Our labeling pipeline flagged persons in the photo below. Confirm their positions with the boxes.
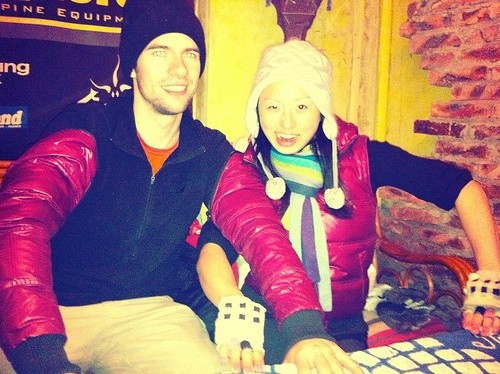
[1,1,362,374]
[195,37,500,374]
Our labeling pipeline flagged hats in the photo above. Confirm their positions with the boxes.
[118,0,209,84]
[247,40,347,209]
